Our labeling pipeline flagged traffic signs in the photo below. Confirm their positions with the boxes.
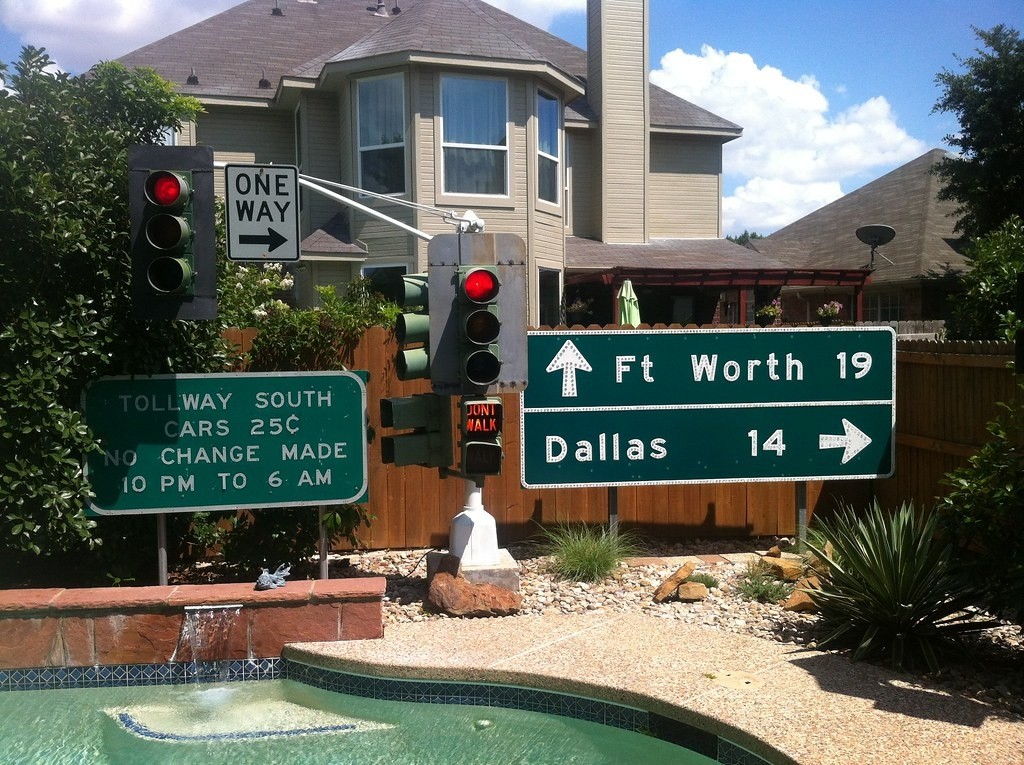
[520,408,897,491]
[520,330,895,406]
[224,162,301,264]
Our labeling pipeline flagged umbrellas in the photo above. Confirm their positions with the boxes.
[617,280,641,327]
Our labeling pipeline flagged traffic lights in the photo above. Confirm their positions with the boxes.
[379,392,452,468]
[142,166,197,300]
[454,264,501,387]
[392,273,433,381]
[458,395,506,479]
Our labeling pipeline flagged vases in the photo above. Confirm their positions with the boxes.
[819,317,833,327]
[758,315,770,327]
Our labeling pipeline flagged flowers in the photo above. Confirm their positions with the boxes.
[817,300,845,315]
[756,299,783,315]
